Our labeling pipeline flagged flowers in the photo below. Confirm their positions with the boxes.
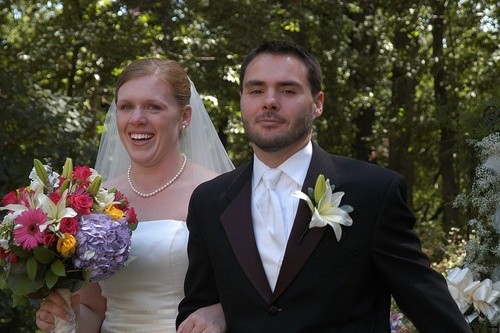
[288,174,355,243]
[0,155,140,332]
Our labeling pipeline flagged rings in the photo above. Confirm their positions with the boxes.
[38,310,43,319]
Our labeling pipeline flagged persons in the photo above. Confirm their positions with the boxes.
[175,38,471,333]
[34,58,236,333]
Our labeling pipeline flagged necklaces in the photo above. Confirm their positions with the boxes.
[127,153,187,198]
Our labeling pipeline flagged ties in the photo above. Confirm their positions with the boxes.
[258,168,287,293]
